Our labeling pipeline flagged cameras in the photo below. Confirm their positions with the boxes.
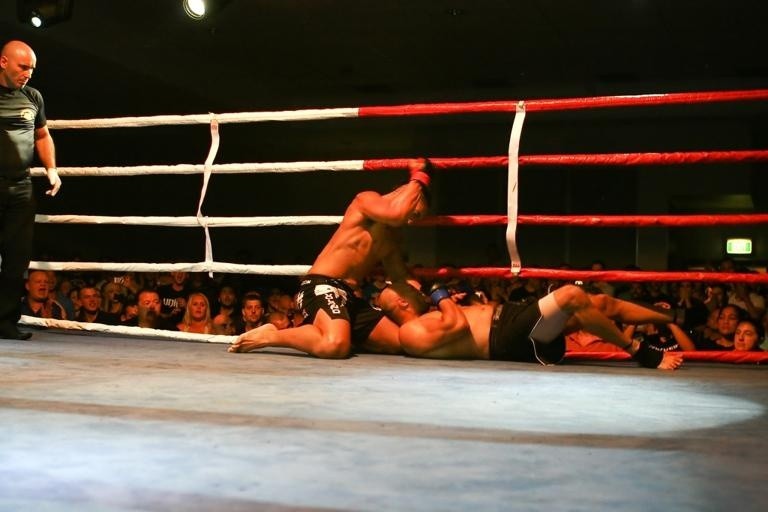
[114,277,124,283]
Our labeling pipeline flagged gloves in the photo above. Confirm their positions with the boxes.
[45,168,62,197]
[423,279,449,306]
[410,157,433,191]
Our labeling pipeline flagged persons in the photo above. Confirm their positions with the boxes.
[225,157,434,362]
[377,279,685,372]
[0,36,62,342]
[0,254,767,354]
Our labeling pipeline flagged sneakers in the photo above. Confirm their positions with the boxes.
[0,329,33,340]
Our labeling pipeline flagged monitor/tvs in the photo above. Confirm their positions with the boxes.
[726,238,753,255]
